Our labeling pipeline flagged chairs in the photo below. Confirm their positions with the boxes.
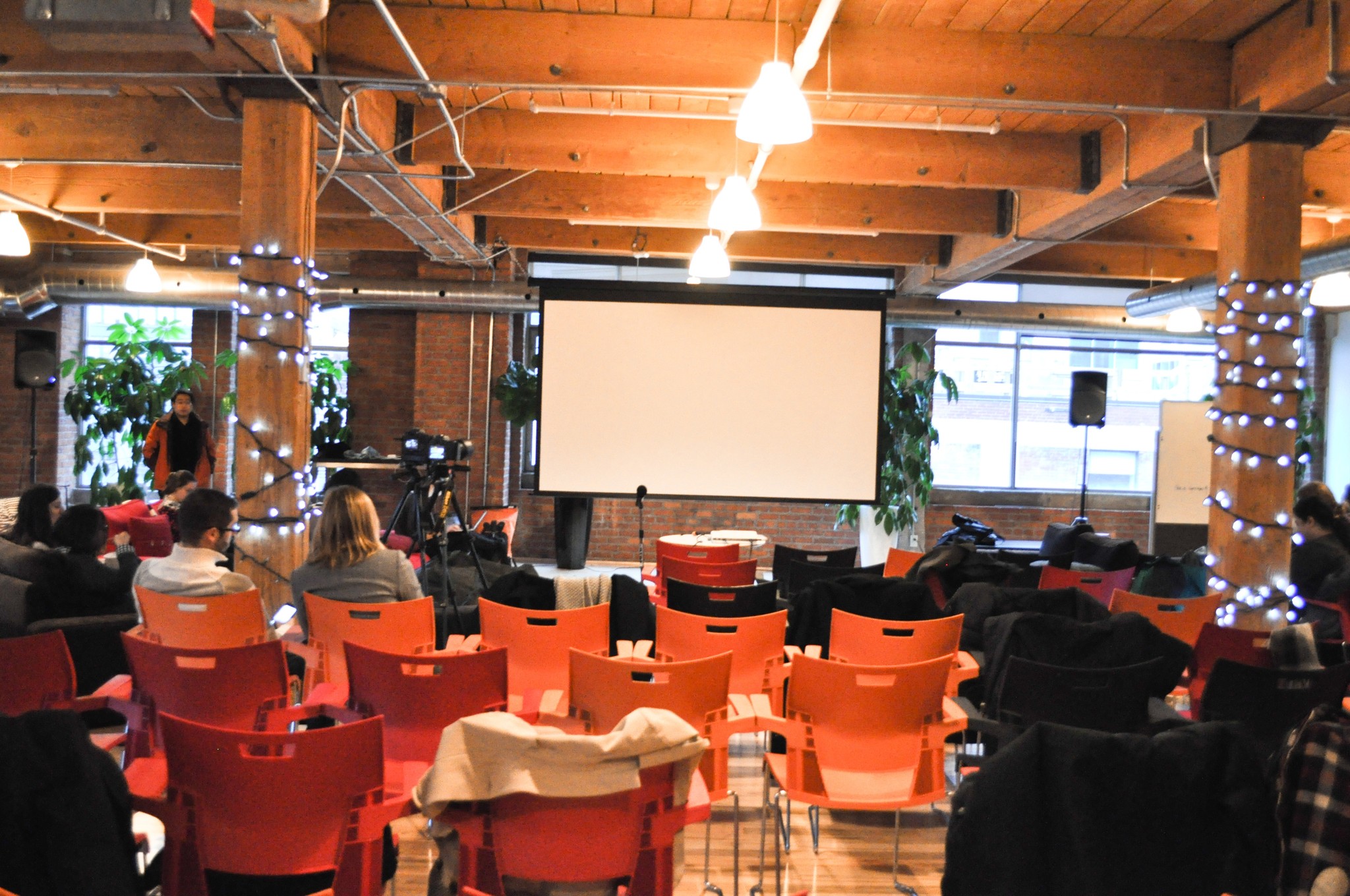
[472,504,522,570]
[1,537,1349,896]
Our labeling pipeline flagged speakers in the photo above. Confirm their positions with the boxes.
[13,328,57,390]
[1068,371,1108,429]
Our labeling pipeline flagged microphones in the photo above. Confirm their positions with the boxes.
[633,485,648,507]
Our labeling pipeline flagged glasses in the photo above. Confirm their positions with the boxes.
[220,524,242,535]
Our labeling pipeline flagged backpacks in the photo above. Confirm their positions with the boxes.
[0,706,148,896]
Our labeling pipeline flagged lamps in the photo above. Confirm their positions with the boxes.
[708,97,765,234]
[126,241,163,293]
[0,158,38,256]
[733,1,814,145]
[689,176,732,279]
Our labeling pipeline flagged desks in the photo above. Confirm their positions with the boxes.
[311,455,461,530]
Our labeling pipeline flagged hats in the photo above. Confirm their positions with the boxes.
[323,467,362,493]
[54,505,109,555]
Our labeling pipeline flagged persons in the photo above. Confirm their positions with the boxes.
[288,488,426,648]
[127,488,281,647]
[49,503,145,624]
[0,485,65,551]
[1290,480,1349,645]
[147,389,219,499]
[1,718,144,894]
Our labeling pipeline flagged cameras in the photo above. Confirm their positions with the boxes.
[397,430,449,465]
[428,438,474,459]
